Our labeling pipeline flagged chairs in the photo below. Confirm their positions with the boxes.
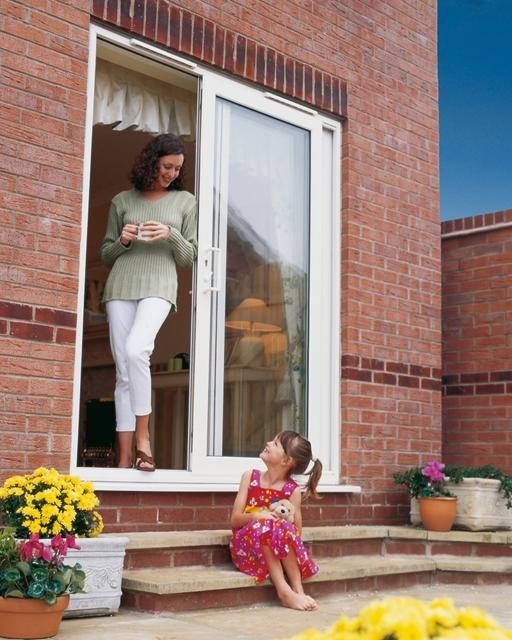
[81,396,120,467]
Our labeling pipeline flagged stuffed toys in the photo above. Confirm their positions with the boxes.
[249,500,296,525]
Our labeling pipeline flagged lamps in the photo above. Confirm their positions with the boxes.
[223,297,285,368]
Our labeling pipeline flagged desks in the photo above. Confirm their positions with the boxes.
[148,367,286,470]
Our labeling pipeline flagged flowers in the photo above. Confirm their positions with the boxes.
[0,463,103,604]
[292,595,511,640]
[420,460,446,496]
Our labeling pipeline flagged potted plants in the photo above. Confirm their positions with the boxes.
[393,461,512,530]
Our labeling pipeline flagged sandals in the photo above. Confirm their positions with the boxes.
[134,445,157,472]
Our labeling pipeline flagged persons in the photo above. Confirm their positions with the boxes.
[96,132,198,473]
[229,427,324,612]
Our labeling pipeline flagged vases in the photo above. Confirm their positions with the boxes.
[0,595,69,640]
[419,495,457,532]
[10,537,130,620]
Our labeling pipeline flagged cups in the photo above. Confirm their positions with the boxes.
[136,223,154,242]
